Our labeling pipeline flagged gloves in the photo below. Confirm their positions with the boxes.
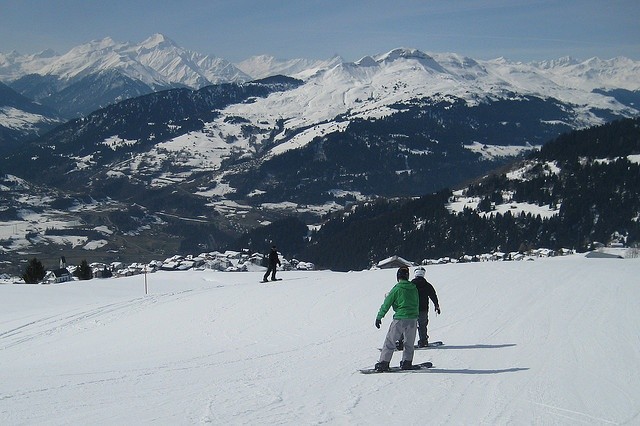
[375,319,382,329]
[434,304,440,313]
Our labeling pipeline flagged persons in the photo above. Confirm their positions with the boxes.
[263,246,281,282]
[399,266,440,349]
[375,267,419,372]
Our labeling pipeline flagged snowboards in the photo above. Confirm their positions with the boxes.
[259,279,282,283]
[377,341,443,351]
[359,362,433,374]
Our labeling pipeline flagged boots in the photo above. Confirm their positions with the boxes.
[418,339,428,348]
[399,360,420,370]
[375,360,390,371]
[397,340,403,349]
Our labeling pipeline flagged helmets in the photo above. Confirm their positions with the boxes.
[396,265,409,282]
[414,266,426,277]
